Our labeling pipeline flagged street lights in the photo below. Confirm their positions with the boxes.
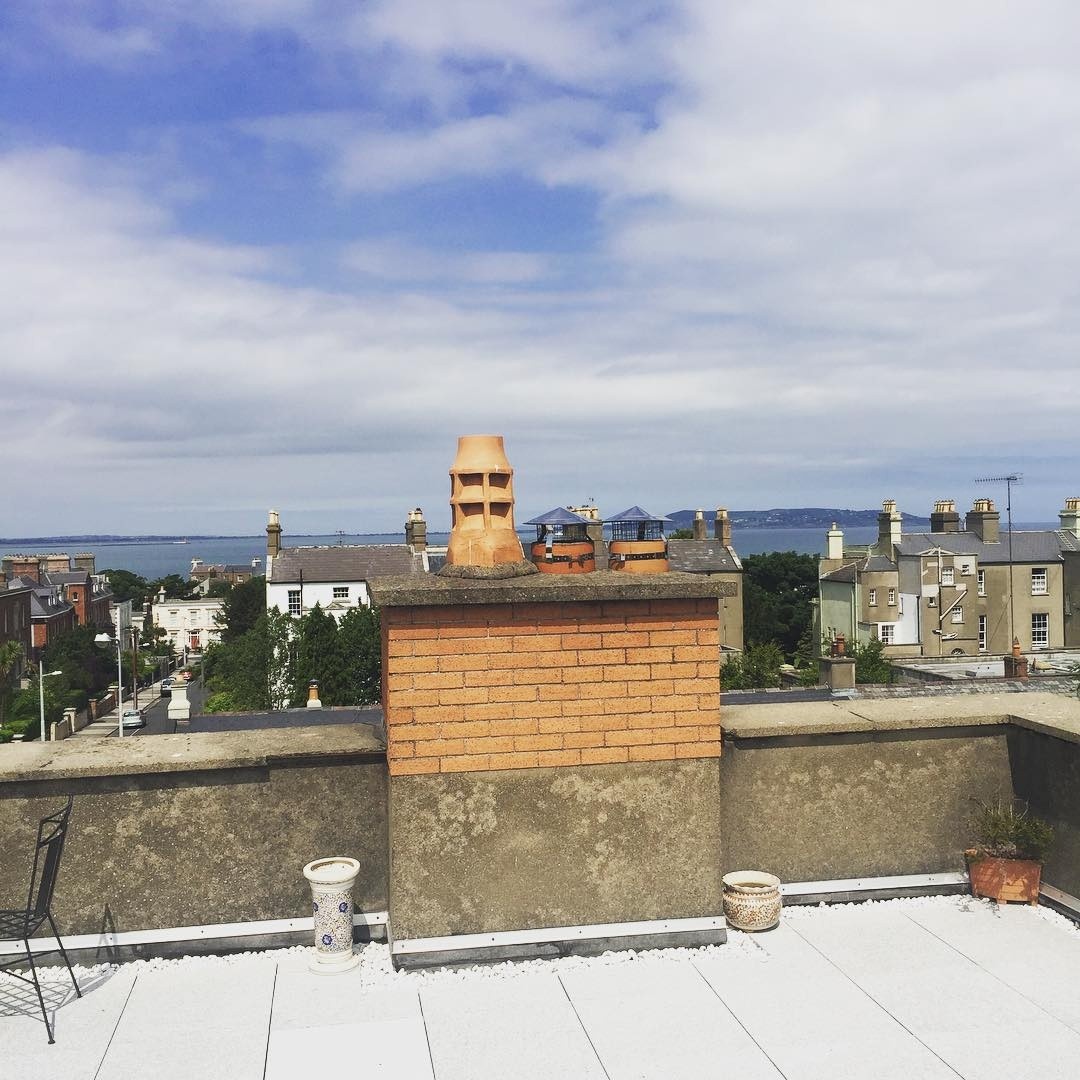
[133,643,150,709]
[94,632,123,737]
[184,617,191,666]
[40,660,62,741]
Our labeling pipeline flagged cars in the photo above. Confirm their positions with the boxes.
[123,710,147,728]
[184,671,191,679]
[161,676,175,690]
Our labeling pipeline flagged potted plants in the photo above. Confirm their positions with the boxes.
[964,795,1056,907]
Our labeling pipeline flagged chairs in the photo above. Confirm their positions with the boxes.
[0,794,82,1044]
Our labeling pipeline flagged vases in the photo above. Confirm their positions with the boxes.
[721,870,782,933]
[303,857,361,976]
